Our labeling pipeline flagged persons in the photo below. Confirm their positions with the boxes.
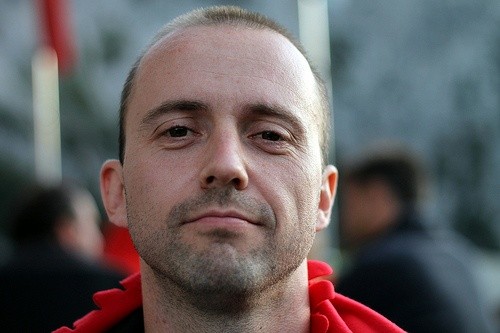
[0,183,134,333]
[53,4,409,333]
[331,147,490,332]
